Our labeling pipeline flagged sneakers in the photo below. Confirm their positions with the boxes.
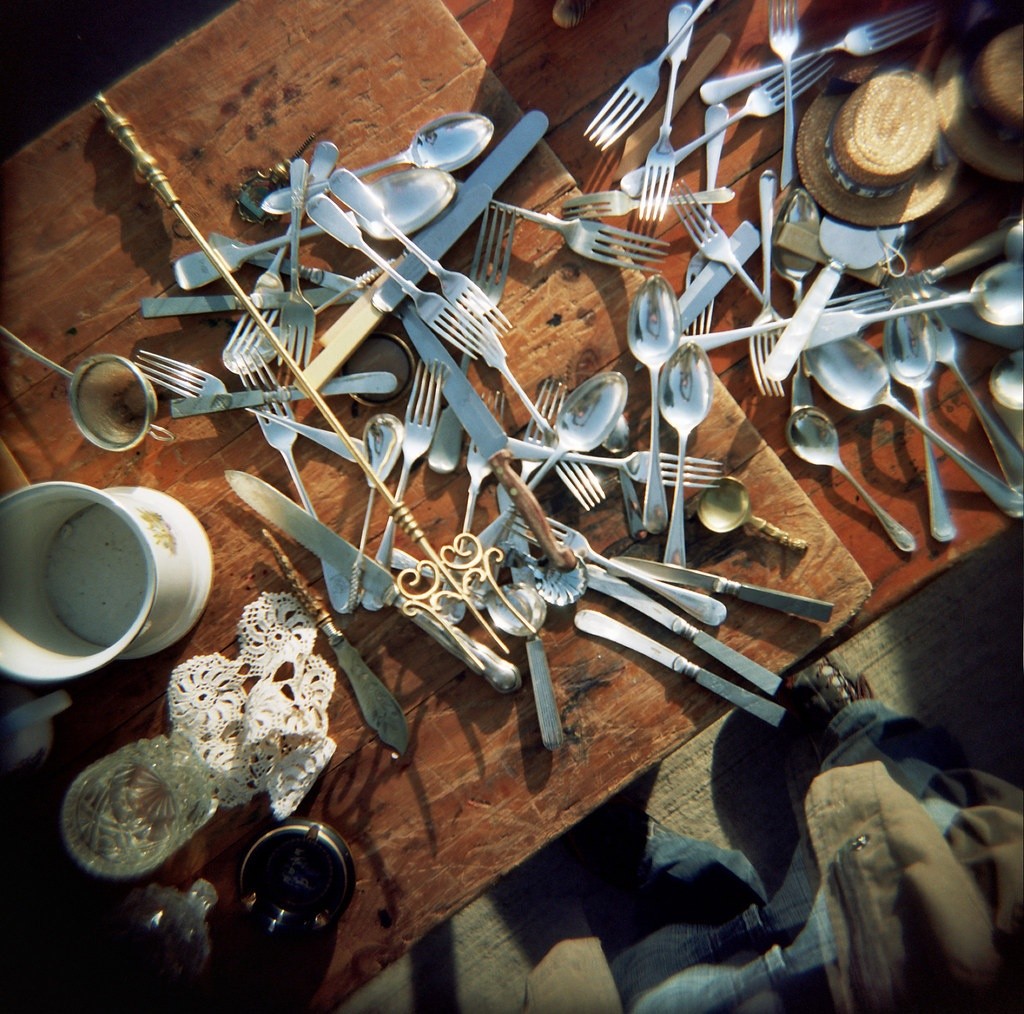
[764,655,872,744]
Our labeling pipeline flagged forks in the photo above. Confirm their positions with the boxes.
[133,0,1023,754]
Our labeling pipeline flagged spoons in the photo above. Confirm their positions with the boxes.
[658,338,715,574]
[623,275,681,536]
[805,338,1024,517]
[261,108,495,218]
[784,406,915,555]
[880,295,956,543]
[380,546,547,641]
[174,172,453,293]
[455,369,630,580]
[801,262,1024,346]
[340,413,406,615]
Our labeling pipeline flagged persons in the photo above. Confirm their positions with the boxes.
[525,646,1024,1012]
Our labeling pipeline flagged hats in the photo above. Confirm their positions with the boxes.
[933,24,1024,182]
[795,65,960,226]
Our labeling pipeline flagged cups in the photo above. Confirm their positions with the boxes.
[0,481,212,682]
[113,881,218,990]
[236,821,353,937]
[62,735,213,877]
